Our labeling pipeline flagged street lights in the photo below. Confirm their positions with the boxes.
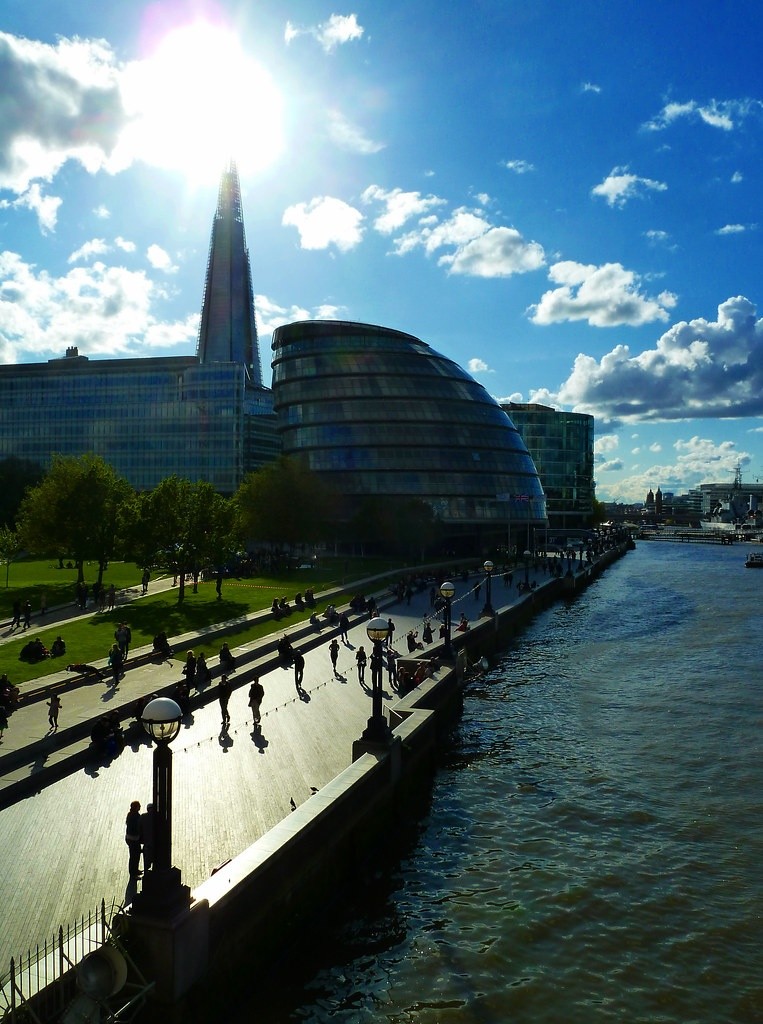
[439,581,455,653]
[586,539,592,562]
[578,541,584,568]
[523,550,530,586]
[139,697,184,895]
[483,561,493,610]
[366,618,389,729]
[595,526,627,552]
[566,544,573,572]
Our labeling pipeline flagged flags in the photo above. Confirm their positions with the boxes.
[496,492,529,505]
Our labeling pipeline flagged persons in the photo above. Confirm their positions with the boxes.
[277,634,294,662]
[272,596,292,615]
[11,588,48,630]
[171,682,192,715]
[304,589,315,604]
[109,620,132,684]
[296,592,305,608]
[0,673,19,738]
[249,677,264,725]
[126,801,154,880]
[59,552,204,615]
[220,642,235,673]
[323,548,593,698]
[217,673,233,724]
[91,709,124,753]
[293,649,304,687]
[185,649,196,684]
[132,693,157,725]
[66,663,106,679]
[153,631,175,657]
[46,693,62,728]
[310,611,322,632]
[20,636,66,660]
[197,652,211,682]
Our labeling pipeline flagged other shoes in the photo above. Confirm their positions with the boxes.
[253,717,261,725]
[137,869,143,874]
[221,716,230,724]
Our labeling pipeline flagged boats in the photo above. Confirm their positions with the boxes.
[744,552,763,568]
[699,458,763,532]
